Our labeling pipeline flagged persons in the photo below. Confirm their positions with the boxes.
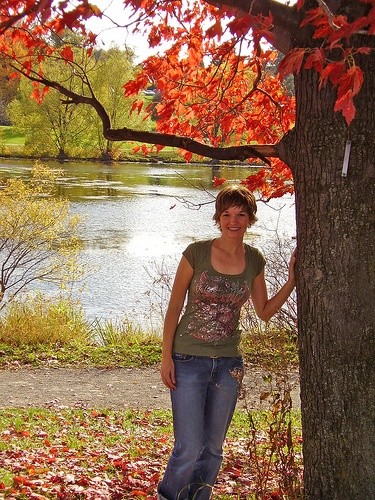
[157,186,300,500]
